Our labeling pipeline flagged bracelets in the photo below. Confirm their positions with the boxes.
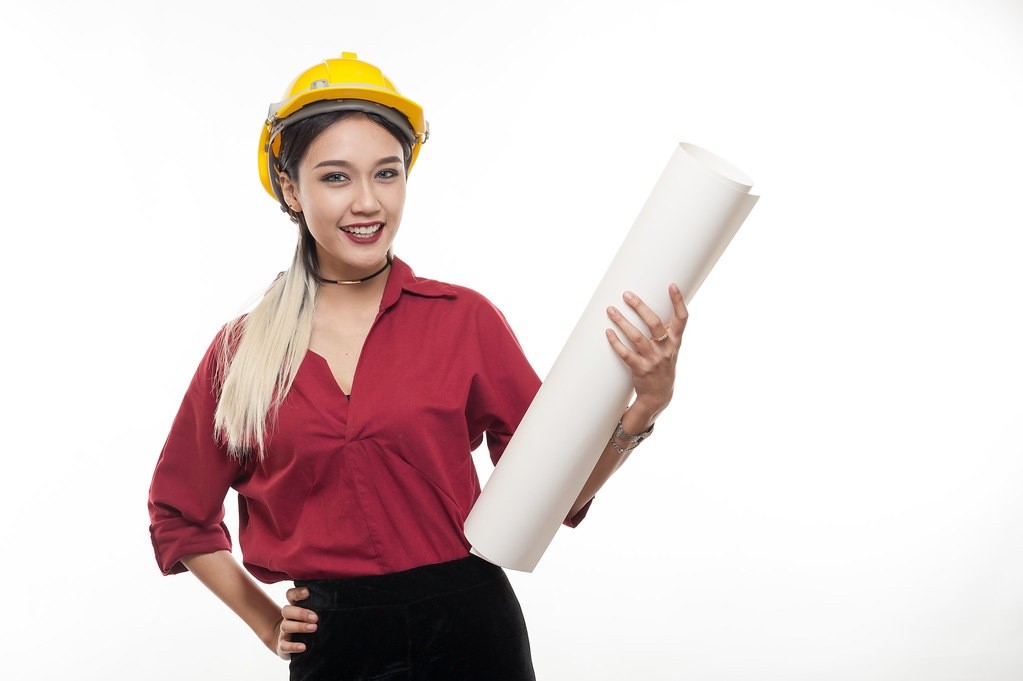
[614,411,654,443]
[610,438,640,453]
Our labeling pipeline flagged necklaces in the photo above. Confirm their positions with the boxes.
[316,260,390,285]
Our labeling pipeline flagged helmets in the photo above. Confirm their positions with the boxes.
[257,52,425,202]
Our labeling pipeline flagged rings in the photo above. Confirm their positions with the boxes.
[652,333,668,342]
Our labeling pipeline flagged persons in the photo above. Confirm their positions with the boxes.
[149,55,688,681]
